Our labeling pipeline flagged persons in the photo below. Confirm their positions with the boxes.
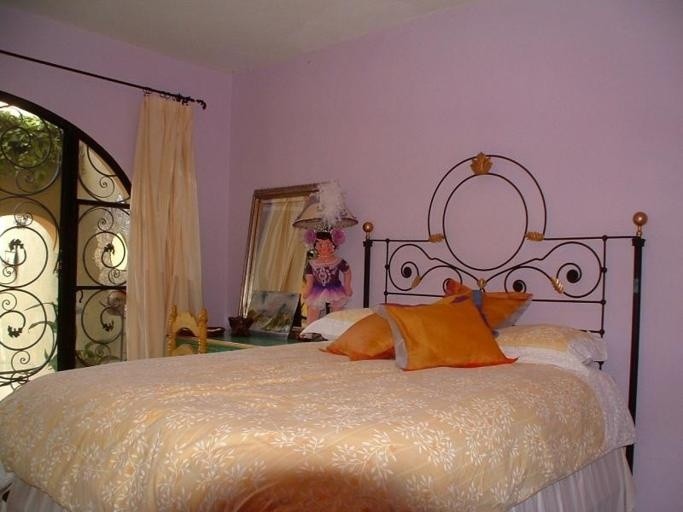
[301,228,352,325]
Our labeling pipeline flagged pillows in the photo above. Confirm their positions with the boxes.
[374,298,519,372]
[327,312,396,361]
[494,324,607,372]
[434,279,532,328]
[300,307,375,342]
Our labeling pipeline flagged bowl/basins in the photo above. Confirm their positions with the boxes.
[229,317,248,332]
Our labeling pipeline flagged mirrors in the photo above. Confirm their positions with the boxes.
[237,181,318,339]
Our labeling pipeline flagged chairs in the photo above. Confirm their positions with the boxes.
[163,303,210,356]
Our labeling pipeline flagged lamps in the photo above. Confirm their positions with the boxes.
[290,181,357,238]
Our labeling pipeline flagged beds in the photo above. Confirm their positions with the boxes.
[0,149,647,512]
